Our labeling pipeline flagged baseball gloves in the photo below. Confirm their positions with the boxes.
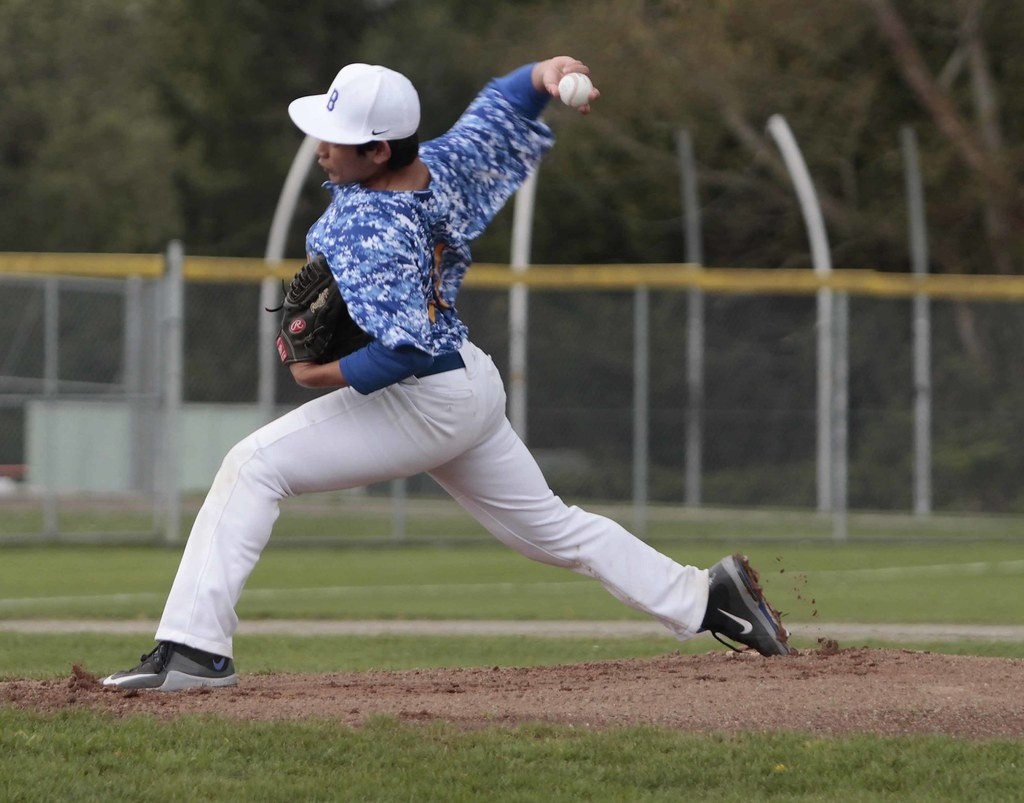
[275,254,349,366]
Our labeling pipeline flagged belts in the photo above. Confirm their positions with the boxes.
[413,351,465,379]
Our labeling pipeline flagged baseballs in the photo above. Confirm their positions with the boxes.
[559,72,594,108]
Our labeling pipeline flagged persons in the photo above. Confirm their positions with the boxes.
[102,54,788,693]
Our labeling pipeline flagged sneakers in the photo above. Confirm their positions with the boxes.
[96,641,237,691]
[696,553,792,656]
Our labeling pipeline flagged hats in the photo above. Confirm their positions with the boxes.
[288,62,420,145]
